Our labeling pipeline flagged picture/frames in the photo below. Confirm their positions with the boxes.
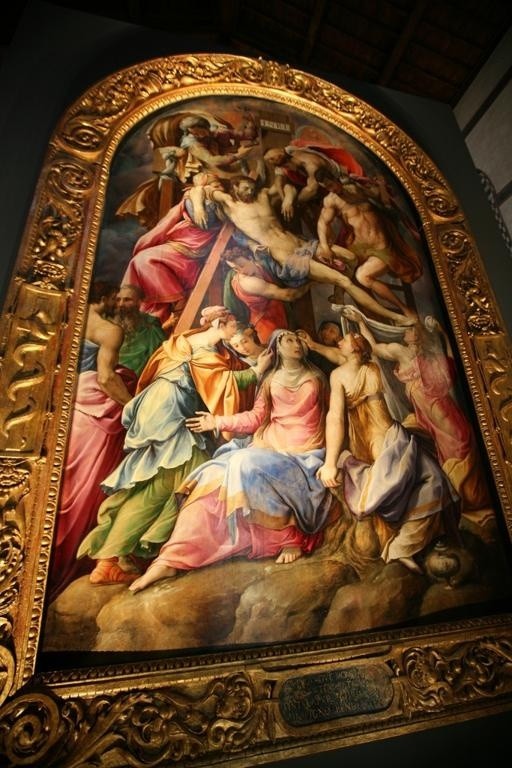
[0,54,512,768]
